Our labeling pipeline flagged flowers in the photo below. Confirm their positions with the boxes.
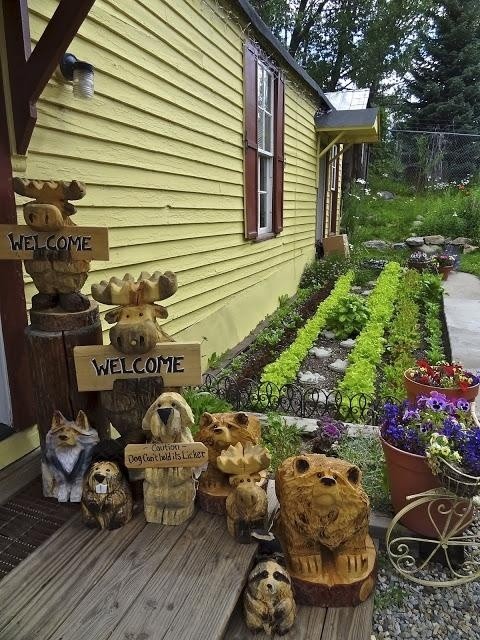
[380,359,480,477]
[408,250,457,267]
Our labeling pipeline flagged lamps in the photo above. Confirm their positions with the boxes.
[59,52,94,100]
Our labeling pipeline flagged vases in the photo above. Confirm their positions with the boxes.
[379,430,473,539]
[408,261,430,274]
[434,265,453,281]
